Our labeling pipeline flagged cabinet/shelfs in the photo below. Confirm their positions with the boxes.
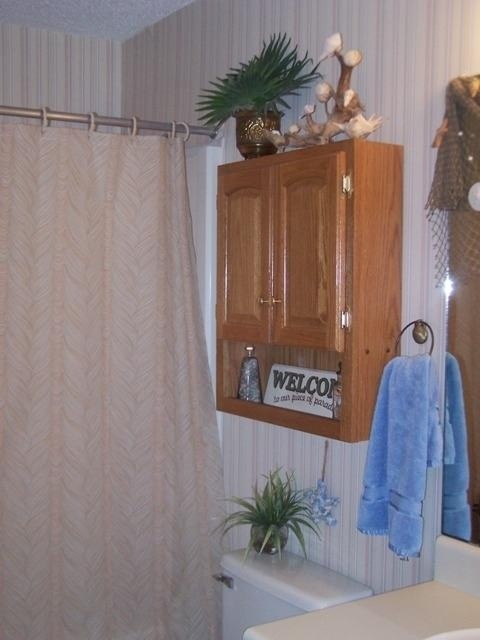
[198,142,399,442]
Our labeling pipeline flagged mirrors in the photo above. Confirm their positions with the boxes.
[443,76,480,548]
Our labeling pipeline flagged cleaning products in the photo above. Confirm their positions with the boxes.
[235,346,263,403]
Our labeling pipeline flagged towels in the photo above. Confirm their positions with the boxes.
[351,355,439,554]
[437,349,471,544]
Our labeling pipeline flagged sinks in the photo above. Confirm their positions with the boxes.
[424,626,480,640]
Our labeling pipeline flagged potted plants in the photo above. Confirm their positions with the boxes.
[194,29,320,158]
[208,471,317,559]
